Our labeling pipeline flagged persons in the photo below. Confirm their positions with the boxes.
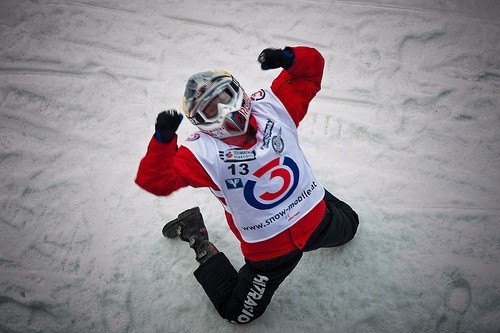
[136,46,360,325]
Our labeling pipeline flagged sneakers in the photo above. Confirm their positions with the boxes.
[163,207,207,246]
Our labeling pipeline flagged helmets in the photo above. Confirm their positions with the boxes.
[184,69,252,138]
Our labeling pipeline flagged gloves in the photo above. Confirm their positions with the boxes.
[258,48,290,70]
[154,110,183,143]
